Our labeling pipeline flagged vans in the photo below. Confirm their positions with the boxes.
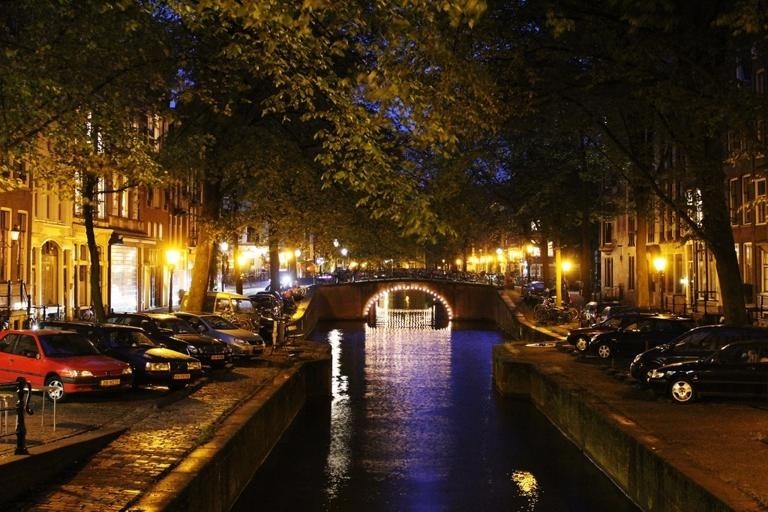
[565,301,767,404]
[180,292,259,323]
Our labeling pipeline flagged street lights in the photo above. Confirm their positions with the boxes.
[561,260,572,299]
[166,249,179,311]
[653,259,667,309]
[333,239,338,269]
[220,243,229,291]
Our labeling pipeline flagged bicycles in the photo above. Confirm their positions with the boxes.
[412,268,485,284]
[82,304,106,320]
[535,297,578,321]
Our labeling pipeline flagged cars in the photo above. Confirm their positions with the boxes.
[0,313,267,404]
[373,268,406,278]
[485,272,504,285]
[250,286,307,314]
[317,266,355,285]
[521,281,545,299]
